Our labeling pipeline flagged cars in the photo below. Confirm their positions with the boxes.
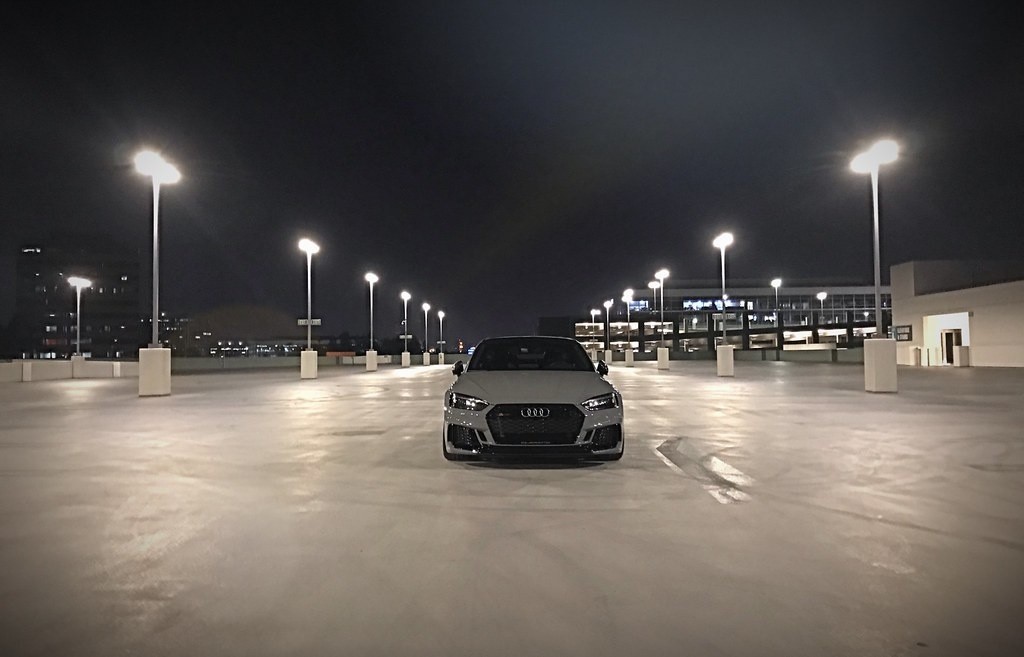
[442,336,625,460]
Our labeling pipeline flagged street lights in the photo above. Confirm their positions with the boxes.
[68,277,91,355]
[621,288,633,349]
[422,303,431,353]
[771,278,782,310]
[297,238,319,351]
[365,272,378,352]
[133,151,179,349]
[849,140,900,337]
[655,269,669,347]
[816,292,827,323]
[401,291,411,351]
[648,282,660,312]
[438,310,445,352]
[590,309,596,350]
[712,231,734,344]
[604,301,612,348]
